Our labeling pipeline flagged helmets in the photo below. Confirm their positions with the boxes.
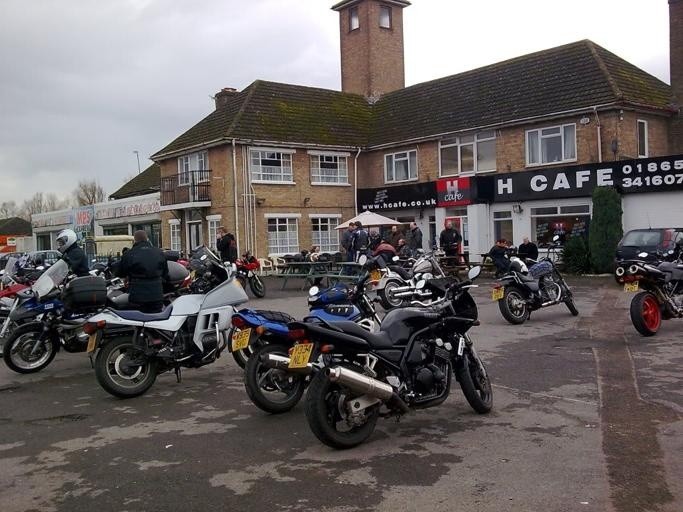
[55,228,77,253]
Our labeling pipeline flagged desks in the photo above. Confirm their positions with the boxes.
[481,252,528,269]
[332,262,361,288]
[280,261,331,290]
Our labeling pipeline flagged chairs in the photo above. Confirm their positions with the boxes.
[257,256,273,276]
[271,256,286,274]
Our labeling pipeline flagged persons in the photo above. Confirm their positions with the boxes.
[32,228,91,285]
[307,220,422,286]
[488,236,538,279]
[111,230,169,313]
[217,226,238,264]
[440,220,462,276]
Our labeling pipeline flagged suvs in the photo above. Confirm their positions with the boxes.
[613,227,683,285]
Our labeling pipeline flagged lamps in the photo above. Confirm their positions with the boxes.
[304,198,310,203]
[512,205,523,213]
[257,198,265,204]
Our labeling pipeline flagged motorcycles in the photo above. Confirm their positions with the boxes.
[492,234,579,324]
[617,228,683,336]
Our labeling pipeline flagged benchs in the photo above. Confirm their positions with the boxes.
[473,263,496,271]
[273,271,359,291]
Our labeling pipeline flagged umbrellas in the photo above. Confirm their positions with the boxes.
[332,210,402,236]
